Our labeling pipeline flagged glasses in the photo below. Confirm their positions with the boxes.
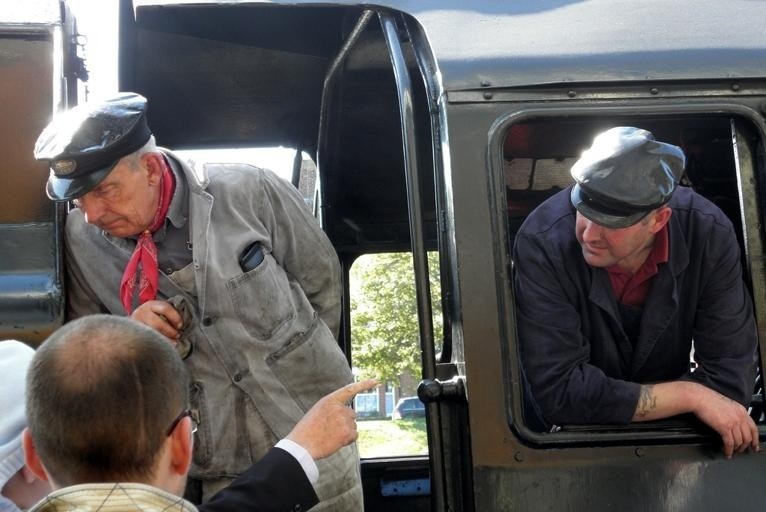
[165,408,203,438]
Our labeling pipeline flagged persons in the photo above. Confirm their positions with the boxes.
[31,91,364,512]
[508,124,762,462]
[20,312,201,512]
[0,340,378,512]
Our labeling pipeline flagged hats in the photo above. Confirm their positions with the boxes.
[571,125,685,229]
[33,92,152,206]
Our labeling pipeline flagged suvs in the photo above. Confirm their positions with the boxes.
[391,395,429,420]
